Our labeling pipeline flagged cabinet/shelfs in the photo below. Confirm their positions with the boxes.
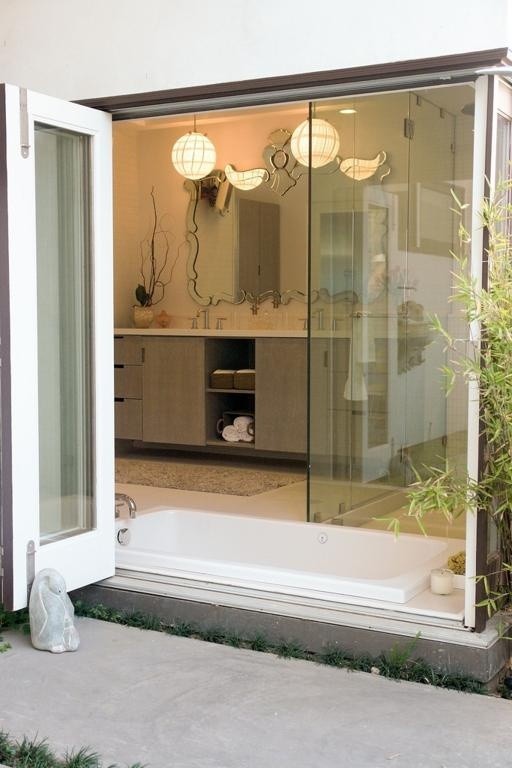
[115,334,142,441]
[309,337,391,463]
[204,337,309,454]
[145,336,207,446]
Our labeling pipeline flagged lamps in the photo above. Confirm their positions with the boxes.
[171,112,217,179]
[289,101,341,169]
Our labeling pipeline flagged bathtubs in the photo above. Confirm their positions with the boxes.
[116,505,465,603]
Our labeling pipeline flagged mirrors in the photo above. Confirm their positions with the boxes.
[182,150,390,306]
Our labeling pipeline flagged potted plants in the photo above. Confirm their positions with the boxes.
[133,284,154,327]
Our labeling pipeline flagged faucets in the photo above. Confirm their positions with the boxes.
[196,309,209,328]
[310,308,323,329]
[115,492,137,518]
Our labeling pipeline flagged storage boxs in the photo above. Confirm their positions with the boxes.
[233,368,256,390]
[210,368,234,389]
